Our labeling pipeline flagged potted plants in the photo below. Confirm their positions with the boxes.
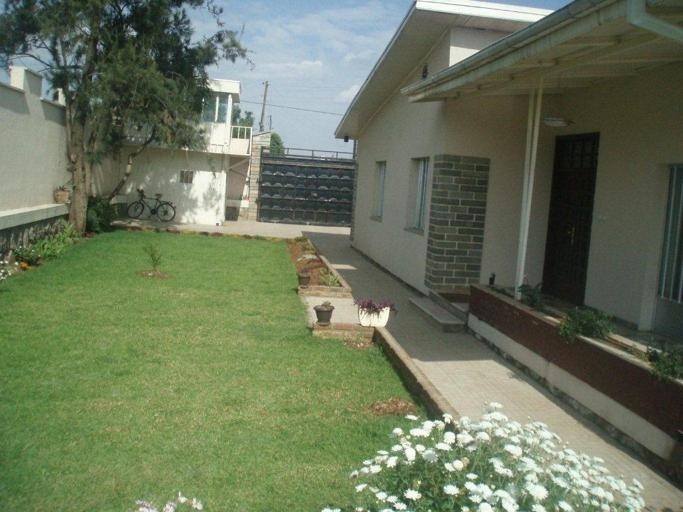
[297,267,311,286]
[313,302,335,325]
[53,185,69,202]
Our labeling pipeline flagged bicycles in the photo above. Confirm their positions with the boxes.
[126,188,176,222]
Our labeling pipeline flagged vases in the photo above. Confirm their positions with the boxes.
[357,308,391,328]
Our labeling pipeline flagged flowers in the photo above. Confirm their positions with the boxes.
[353,297,396,314]
[354,398,651,512]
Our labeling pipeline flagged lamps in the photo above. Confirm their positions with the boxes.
[542,71,575,127]
